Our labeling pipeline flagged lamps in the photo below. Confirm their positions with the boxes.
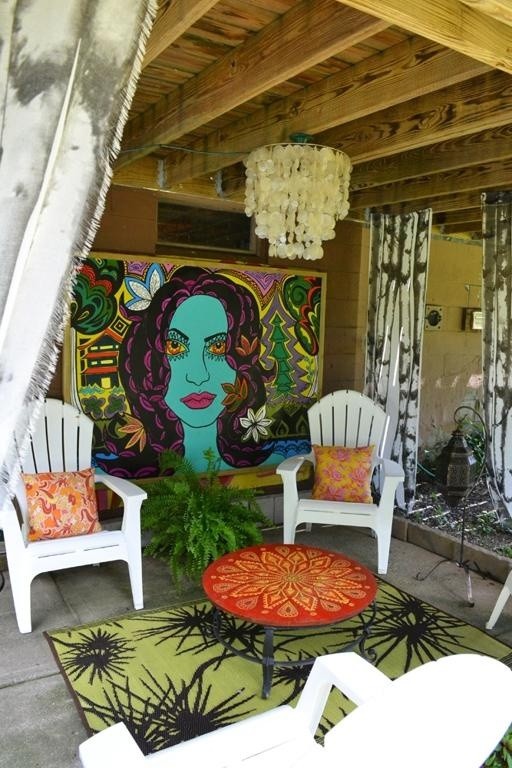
[241,131,355,263]
[417,405,491,607]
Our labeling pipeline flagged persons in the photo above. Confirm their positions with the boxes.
[104,265,288,476]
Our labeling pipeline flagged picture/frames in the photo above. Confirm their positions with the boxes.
[60,252,329,494]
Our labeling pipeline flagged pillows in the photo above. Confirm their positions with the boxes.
[309,443,377,504]
[21,466,102,546]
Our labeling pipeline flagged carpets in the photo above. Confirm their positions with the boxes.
[41,577,512,768]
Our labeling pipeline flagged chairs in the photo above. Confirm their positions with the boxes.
[0,396,148,636]
[273,390,406,575]
[73,654,512,768]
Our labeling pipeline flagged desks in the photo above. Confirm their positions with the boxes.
[203,545,377,702]
